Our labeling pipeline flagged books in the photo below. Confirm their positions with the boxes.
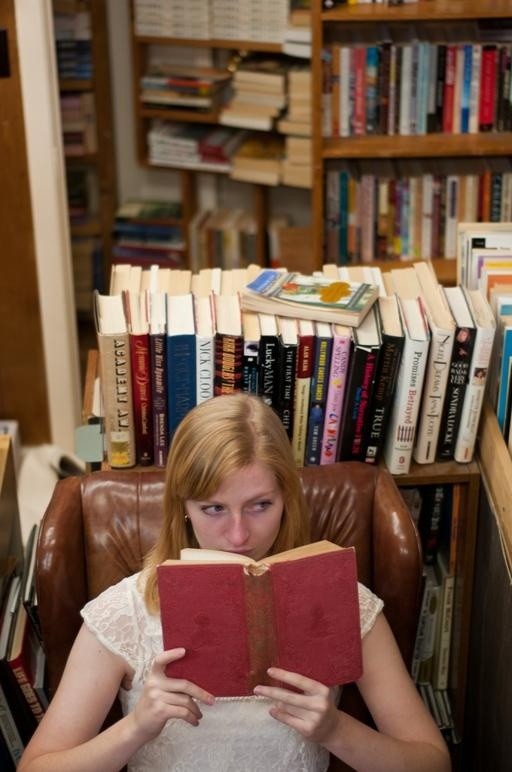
[156,538,364,697]
[111,201,287,273]
[326,170,512,264]
[320,41,512,137]
[134,0,311,190]
[0,522,51,772]
[456,222,512,452]
[93,258,497,476]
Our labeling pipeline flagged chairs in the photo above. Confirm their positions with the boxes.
[36,459,422,766]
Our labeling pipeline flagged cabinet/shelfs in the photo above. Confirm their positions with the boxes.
[92,0,310,271]
[312,2,510,289]
[484,402,512,562]
[82,342,482,749]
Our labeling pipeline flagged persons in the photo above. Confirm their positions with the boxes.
[16,394,452,771]
